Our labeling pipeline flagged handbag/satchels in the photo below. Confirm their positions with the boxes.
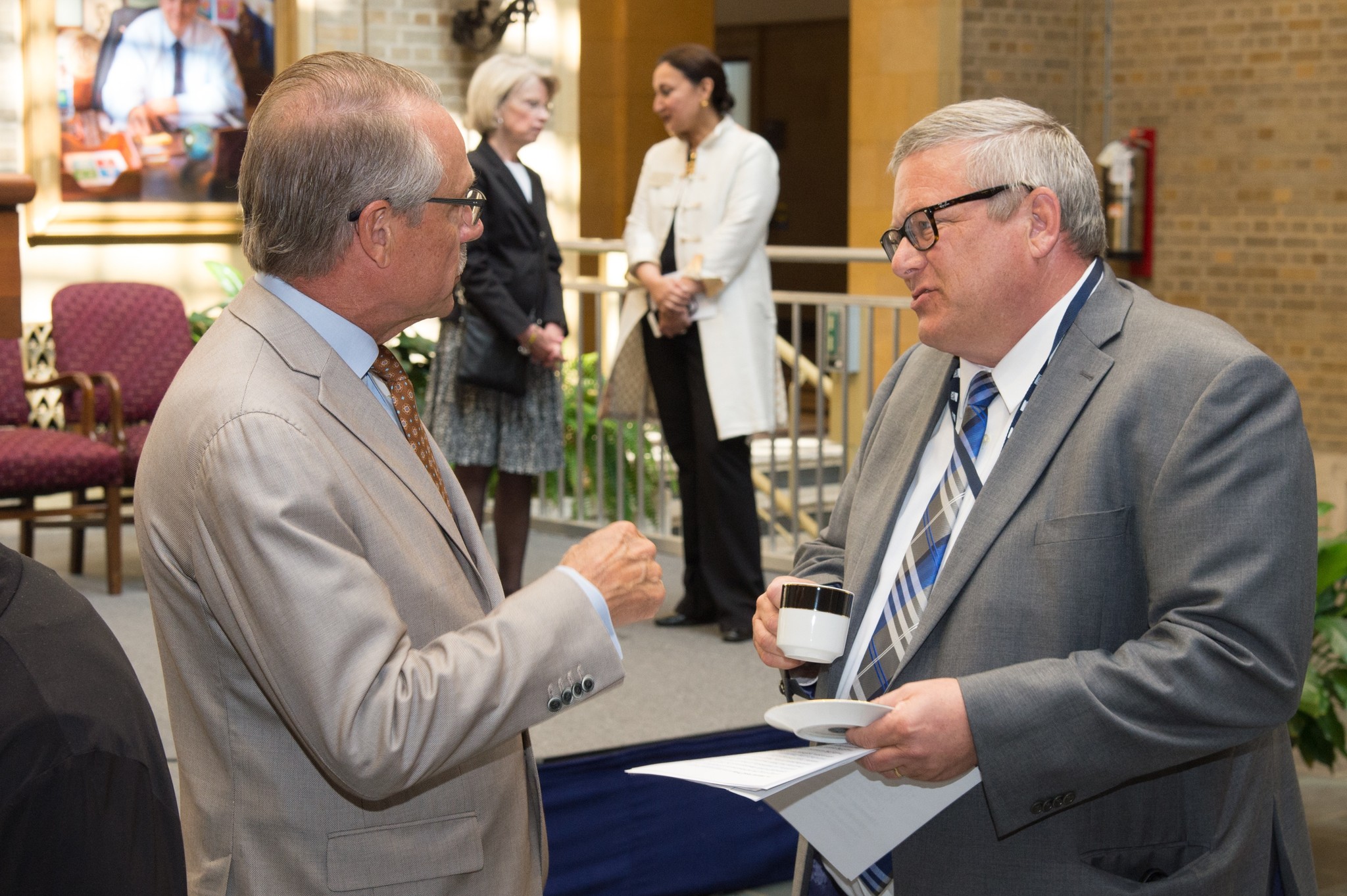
[454,292,532,397]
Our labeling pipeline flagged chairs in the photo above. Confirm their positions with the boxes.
[0,337,124,593]
[51,282,192,575]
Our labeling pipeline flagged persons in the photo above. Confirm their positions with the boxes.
[751,96,1322,896]
[0,540,187,896]
[424,51,574,596]
[595,45,791,655]
[101,0,249,138]
[147,53,668,896]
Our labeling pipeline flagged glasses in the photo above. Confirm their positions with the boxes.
[347,186,487,227]
[880,181,1034,265]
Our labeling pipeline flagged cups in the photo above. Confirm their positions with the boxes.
[775,582,855,664]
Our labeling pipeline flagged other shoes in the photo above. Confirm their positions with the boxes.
[656,612,712,626]
[723,626,753,641]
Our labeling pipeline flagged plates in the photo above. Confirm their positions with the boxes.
[763,699,895,744]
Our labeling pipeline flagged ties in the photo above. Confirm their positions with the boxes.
[372,343,455,517]
[848,370,999,703]
